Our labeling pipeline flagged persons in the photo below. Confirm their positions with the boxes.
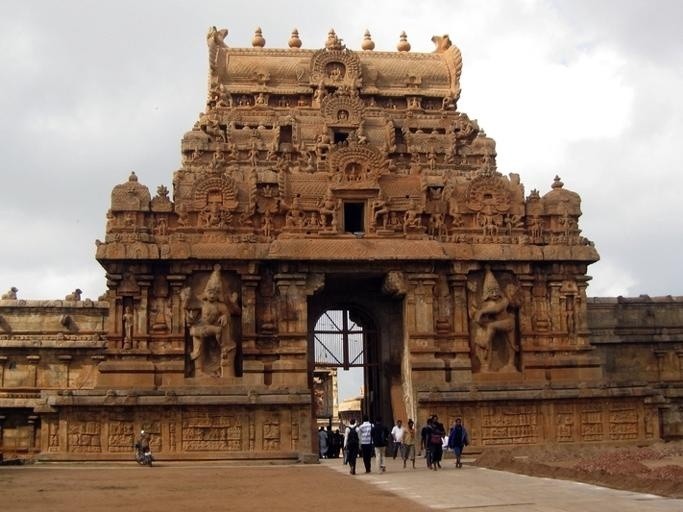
[172,82,518,244]
[136,429,154,457]
[157,213,166,237]
[472,269,517,373]
[318,413,470,477]
[564,301,576,335]
[147,213,155,234]
[559,209,574,238]
[120,304,134,341]
[181,268,231,364]
[114,231,120,239]
[122,212,137,234]
[528,211,543,239]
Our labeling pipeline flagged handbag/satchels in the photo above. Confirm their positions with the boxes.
[431,434,441,444]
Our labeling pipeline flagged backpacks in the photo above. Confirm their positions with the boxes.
[348,426,358,449]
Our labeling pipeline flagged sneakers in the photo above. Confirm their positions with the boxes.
[456,462,462,467]
[427,463,441,470]
[351,464,386,474]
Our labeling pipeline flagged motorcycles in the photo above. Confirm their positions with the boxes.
[131,440,155,468]
